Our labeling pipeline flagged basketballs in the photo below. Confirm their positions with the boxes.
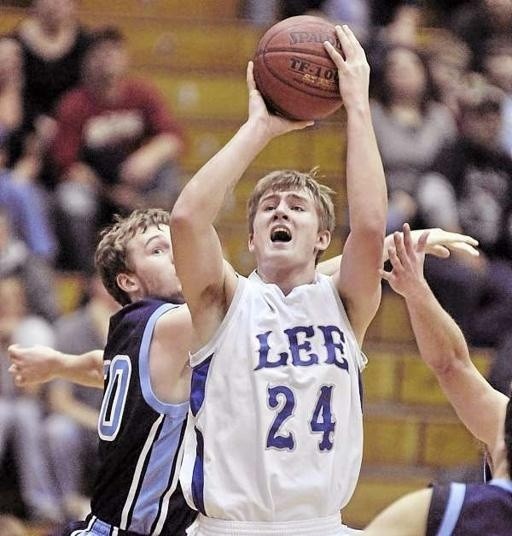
[254,15,344,120]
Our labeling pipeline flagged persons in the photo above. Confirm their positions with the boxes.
[1,0,512,536]
